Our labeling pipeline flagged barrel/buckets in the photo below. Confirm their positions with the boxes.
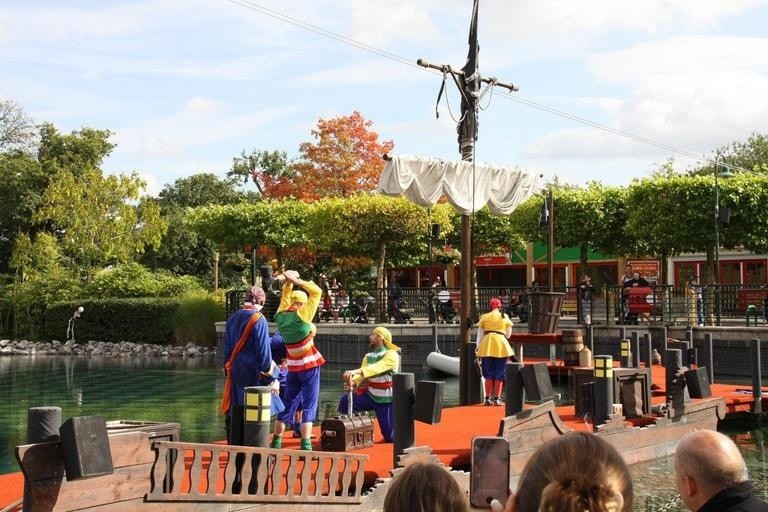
[562,328,585,367]
[579,345,592,367]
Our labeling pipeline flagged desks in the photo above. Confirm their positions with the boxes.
[510,331,563,364]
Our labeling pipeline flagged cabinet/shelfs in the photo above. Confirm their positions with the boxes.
[737,288,767,316]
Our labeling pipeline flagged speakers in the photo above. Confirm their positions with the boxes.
[413,380,446,425]
[432,224,440,237]
[520,363,555,403]
[684,366,711,399]
[720,208,731,224]
[59,415,113,482]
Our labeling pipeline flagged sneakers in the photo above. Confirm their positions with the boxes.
[485,401,493,405]
[494,402,504,406]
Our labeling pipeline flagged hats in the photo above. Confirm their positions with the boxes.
[290,291,307,303]
[245,287,266,305]
[488,298,501,309]
[373,327,400,351]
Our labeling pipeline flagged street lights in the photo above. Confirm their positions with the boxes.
[712,150,733,326]
[543,187,549,223]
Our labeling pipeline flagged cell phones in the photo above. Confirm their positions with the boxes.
[470,437,510,509]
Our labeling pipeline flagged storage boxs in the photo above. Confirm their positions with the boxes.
[320,415,375,452]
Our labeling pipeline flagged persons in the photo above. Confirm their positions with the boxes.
[674,430,768,512]
[221,271,349,491]
[383,462,467,512]
[336,327,401,442]
[497,280,540,322]
[388,277,402,324]
[431,275,440,314]
[579,277,595,324]
[626,271,648,324]
[474,298,516,406]
[490,433,633,512]
[688,275,704,326]
[621,265,635,322]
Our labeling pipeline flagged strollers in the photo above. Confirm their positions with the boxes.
[350,295,372,325]
[392,299,416,326]
[430,294,459,324]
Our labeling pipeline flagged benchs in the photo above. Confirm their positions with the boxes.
[319,298,459,323]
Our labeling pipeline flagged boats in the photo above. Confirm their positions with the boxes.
[428,351,564,380]
